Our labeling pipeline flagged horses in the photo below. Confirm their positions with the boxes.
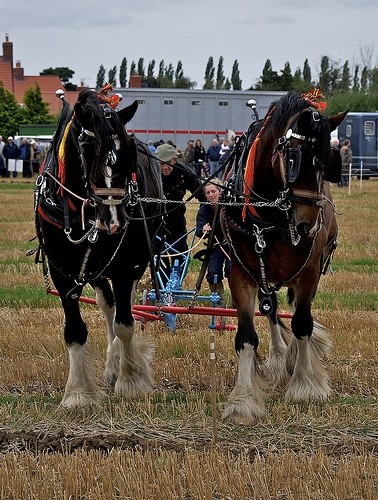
[217,89,352,420]
[33,90,163,410]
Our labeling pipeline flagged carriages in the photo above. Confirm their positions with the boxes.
[33,82,353,429]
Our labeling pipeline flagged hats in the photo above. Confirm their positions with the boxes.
[155,143,177,162]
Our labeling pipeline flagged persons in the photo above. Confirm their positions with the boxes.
[148,140,176,153]
[219,141,231,177]
[332,139,353,187]
[0,135,6,178]
[196,178,234,309]
[152,143,206,290]
[207,138,221,178]
[176,150,186,165]
[19,137,32,179]
[184,140,194,171]
[29,141,40,176]
[195,139,205,177]
[2,136,20,178]
